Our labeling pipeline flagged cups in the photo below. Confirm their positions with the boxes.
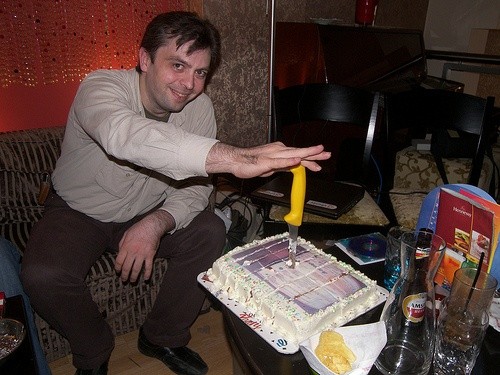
[384,227,416,294]
[442,268,498,353]
[434,297,489,375]
[355,0,379,27]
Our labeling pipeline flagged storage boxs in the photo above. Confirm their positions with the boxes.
[465,28,500,108]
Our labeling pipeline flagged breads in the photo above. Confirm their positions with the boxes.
[316,330,357,374]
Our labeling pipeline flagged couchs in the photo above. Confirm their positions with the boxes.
[0,125,229,361]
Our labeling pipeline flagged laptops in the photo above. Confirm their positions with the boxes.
[249,172,365,221]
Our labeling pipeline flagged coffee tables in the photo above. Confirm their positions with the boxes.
[220,220,500,375]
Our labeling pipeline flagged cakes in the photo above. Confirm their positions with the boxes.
[207,232,380,343]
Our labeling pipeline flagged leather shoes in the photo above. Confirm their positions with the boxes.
[137,326,209,375]
[75,346,115,375]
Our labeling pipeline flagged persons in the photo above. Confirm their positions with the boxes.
[22,10,331,375]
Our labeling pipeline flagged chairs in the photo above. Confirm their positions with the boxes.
[428,97,495,187]
[277,83,399,225]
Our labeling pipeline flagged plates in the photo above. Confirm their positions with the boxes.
[481,298,500,332]
[309,18,345,24]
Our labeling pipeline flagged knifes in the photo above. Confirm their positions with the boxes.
[284,166,307,270]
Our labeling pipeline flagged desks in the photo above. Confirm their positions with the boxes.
[0,295,43,375]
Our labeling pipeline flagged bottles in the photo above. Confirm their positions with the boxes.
[396,228,434,345]
[373,231,447,375]
